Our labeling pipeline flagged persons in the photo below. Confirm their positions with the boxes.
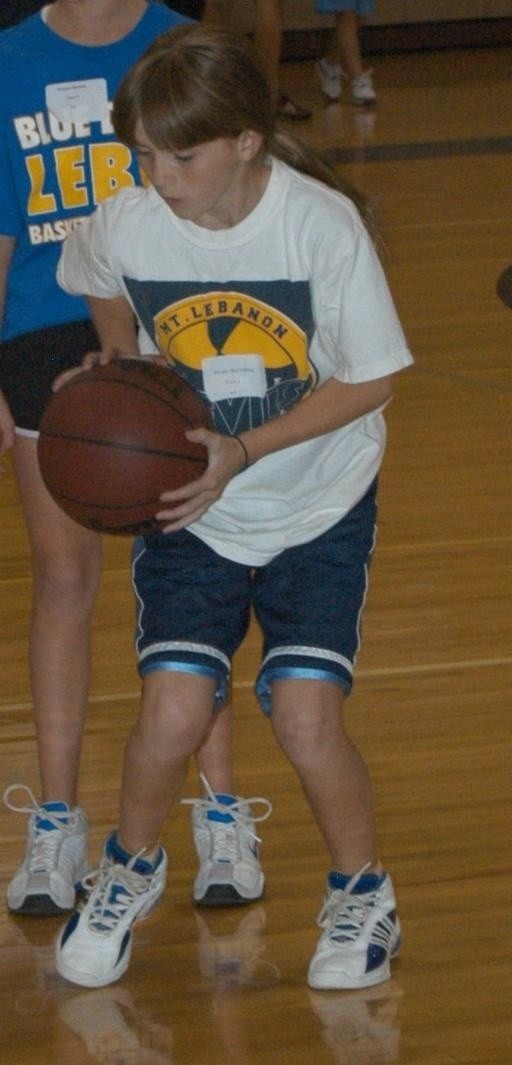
[49,29,412,994]
[312,0,381,103]
[0,0,266,916]
[165,0,314,121]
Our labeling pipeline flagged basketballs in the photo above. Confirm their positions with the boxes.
[37,359,214,536]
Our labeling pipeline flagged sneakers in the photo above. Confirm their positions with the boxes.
[178,770,275,905]
[306,858,403,991]
[2,782,92,916]
[277,94,313,120]
[352,75,376,101]
[53,827,168,989]
[313,57,343,99]
